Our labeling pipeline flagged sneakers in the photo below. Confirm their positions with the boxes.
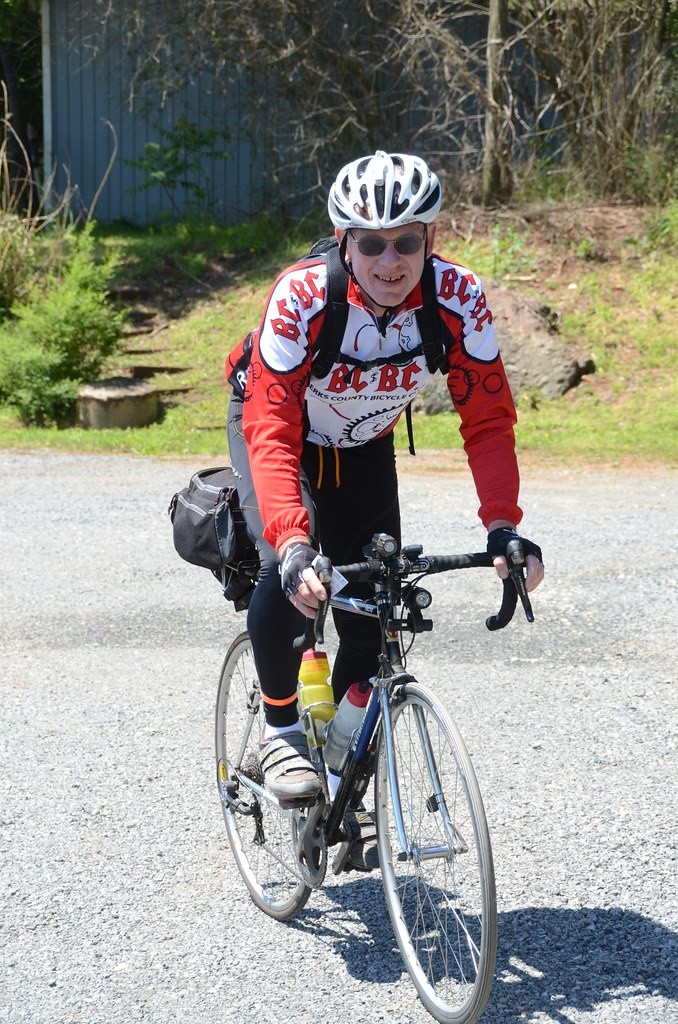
[258,725,321,800]
[330,800,392,868]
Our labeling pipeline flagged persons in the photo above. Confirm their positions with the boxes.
[226,152,546,869]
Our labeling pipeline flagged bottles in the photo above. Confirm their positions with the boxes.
[298,648,336,748]
[322,681,372,771]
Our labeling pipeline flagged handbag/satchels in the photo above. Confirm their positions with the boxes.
[167,466,257,569]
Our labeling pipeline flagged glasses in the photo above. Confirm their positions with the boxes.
[348,229,426,257]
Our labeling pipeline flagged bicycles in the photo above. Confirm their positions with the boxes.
[215,534,536,1024]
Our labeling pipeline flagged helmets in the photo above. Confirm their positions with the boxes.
[327,150,442,230]
